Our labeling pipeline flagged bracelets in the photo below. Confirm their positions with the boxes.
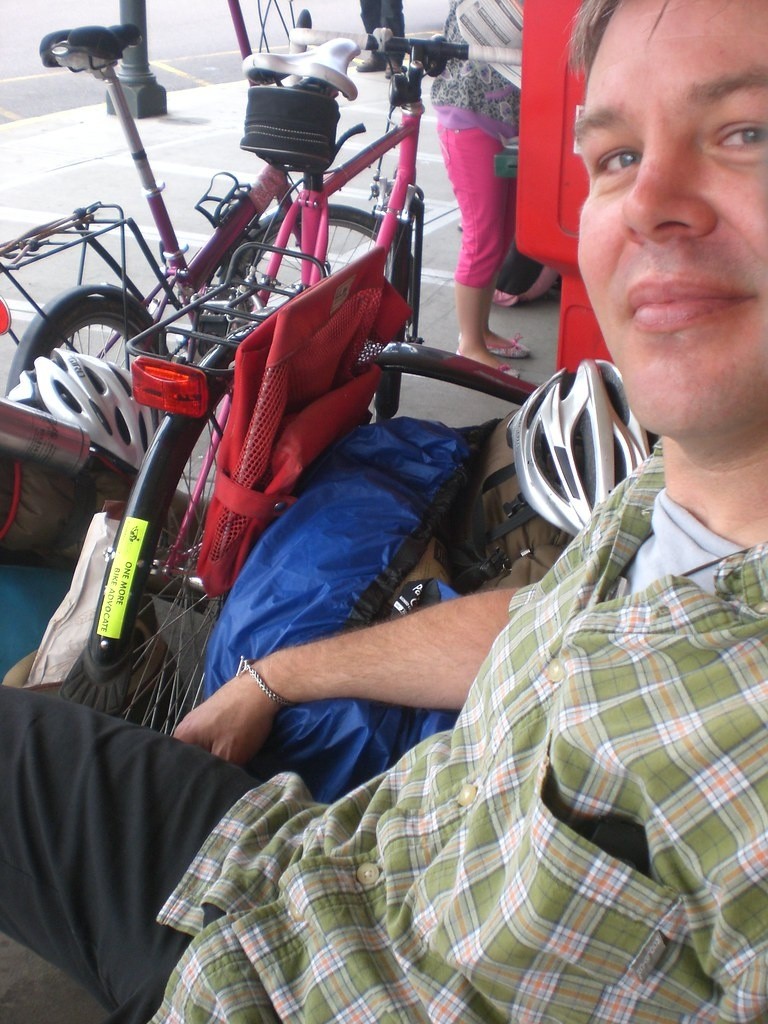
[236,655,292,708]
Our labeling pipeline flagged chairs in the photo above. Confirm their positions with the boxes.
[202,341,539,795]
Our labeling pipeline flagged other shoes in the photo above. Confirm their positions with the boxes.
[455,349,520,379]
[458,330,531,359]
[385,56,403,79]
[356,53,387,73]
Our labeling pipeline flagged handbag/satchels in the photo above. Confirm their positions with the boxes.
[1,449,213,616]
[205,415,506,807]
[240,86,341,173]
[463,407,576,591]
[193,246,413,596]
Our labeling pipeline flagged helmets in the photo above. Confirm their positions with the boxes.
[8,345,170,481]
[505,359,657,539]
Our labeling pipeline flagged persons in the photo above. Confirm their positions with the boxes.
[0,0,768,1024]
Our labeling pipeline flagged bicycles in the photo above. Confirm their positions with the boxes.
[0,20,469,719]
